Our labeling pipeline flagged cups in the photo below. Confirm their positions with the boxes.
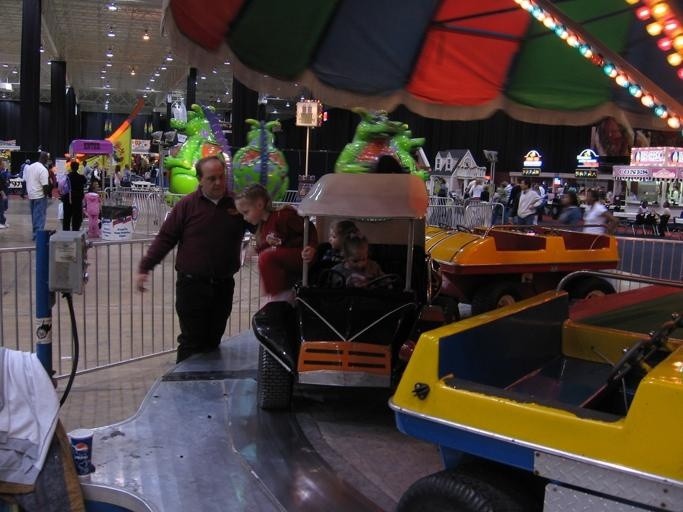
[69,426,94,474]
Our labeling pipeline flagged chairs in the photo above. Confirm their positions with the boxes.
[613,212,683,237]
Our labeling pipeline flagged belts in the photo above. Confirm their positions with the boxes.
[184,271,233,285]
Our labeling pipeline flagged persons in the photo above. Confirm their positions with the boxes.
[320,219,359,271]
[431,169,681,246]
[0,133,131,242]
[135,157,258,364]
[235,185,318,302]
[332,232,389,288]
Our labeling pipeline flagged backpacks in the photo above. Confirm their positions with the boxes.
[57,173,71,196]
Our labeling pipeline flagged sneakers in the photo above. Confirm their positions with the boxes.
[0,223,9,229]
